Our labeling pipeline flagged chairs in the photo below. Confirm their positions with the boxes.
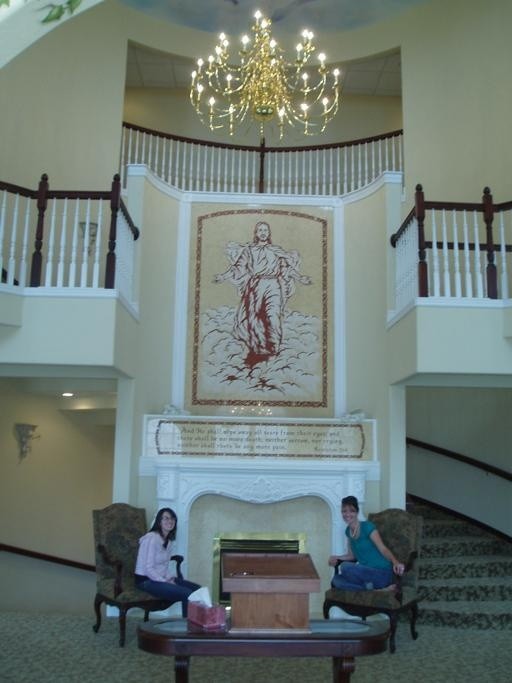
[92,500,185,647]
[323,508,425,653]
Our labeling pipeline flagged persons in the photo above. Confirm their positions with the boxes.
[131,508,201,617]
[327,495,406,593]
[211,217,315,362]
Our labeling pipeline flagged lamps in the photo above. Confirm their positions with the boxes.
[189,0,343,140]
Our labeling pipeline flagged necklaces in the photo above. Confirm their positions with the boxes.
[348,522,359,538]
